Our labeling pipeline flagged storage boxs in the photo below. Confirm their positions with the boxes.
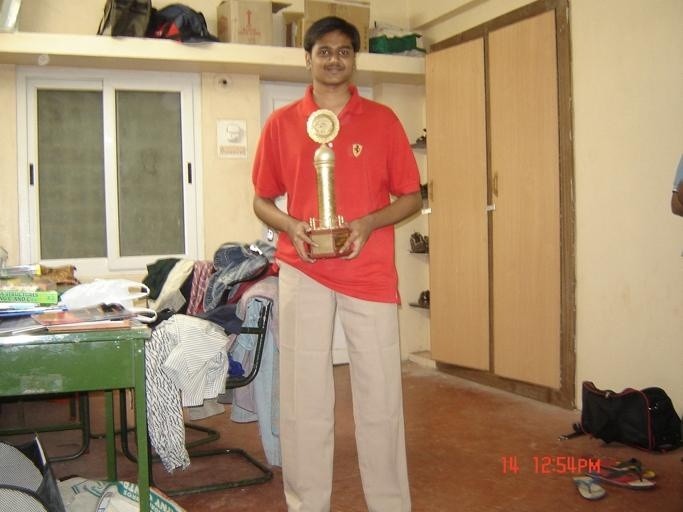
[215,0,369,52]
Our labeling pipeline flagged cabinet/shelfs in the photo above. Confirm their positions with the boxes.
[424,10,564,393]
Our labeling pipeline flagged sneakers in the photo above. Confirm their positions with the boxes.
[419,291,430,306]
[410,233,426,253]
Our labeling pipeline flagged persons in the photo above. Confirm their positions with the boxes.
[250,15,422,511]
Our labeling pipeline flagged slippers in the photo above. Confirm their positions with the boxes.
[572,458,657,500]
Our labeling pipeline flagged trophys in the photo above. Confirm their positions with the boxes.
[300,108,351,259]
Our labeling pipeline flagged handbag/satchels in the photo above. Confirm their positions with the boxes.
[149,4,217,42]
[582,381,683,453]
[96,0,152,37]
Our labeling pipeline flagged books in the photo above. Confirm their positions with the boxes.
[0,286,143,330]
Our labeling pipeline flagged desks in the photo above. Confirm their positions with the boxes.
[0,304,152,512]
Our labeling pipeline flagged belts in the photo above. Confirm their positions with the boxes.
[212,242,269,286]
